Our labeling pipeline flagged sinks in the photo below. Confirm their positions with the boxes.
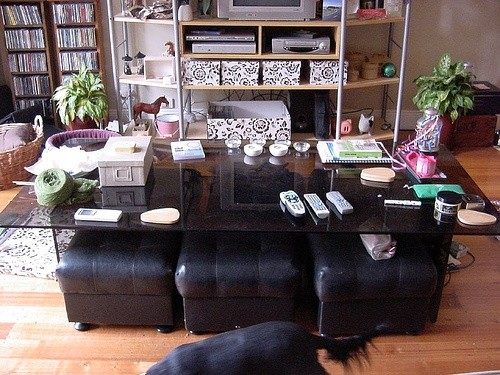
[309,164,438,337]
[175,170,307,336]
[52,166,199,334]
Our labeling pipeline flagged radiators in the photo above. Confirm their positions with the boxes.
[303,199,328,225]
[74,220,122,227]
[303,193,330,219]
[74,208,122,222]
[326,191,354,215]
[326,197,353,221]
[280,201,306,228]
[279,190,305,217]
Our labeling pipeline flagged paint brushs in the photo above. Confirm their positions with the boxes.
[449,240,468,259]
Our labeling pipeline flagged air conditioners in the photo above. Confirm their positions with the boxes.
[144,321,392,375]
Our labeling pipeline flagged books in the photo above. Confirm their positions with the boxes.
[452,115,497,150]
[308,60,349,85]
[182,59,221,86]
[206,99,293,141]
[261,60,301,86]
[97,136,154,186]
[222,59,260,86]
[468,80,500,115]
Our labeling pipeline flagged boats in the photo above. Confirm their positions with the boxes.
[287,91,314,133]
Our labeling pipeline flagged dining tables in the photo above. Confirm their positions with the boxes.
[411,51,477,152]
[51,60,108,131]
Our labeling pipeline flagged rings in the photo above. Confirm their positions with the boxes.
[384,199,432,206]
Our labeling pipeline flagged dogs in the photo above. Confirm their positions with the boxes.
[134,95,169,131]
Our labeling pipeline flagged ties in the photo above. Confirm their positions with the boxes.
[219,147,310,212]
[216,0,316,22]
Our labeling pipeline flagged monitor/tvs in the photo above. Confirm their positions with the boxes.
[316,140,393,164]
[12,76,50,96]
[15,98,53,111]
[324,165,391,175]
[59,51,99,71]
[0,4,43,25]
[57,27,97,49]
[170,140,206,160]
[8,52,48,73]
[62,73,101,87]
[4,28,45,49]
[52,3,95,24]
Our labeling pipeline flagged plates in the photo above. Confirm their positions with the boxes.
[107,0,411,154]
[0,0,60,127]
[49,0,110,128]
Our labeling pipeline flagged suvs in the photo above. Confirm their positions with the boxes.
[153,115,179,139]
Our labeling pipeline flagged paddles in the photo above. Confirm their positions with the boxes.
[0,175,78,284]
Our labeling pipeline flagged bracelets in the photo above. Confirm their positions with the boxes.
[249,138,266,146]
[294,142,310,152]
[275,139,291,148]
[244,144,263,157]
[243,155,263,165]
[225,138,242,148]
[268,144,288,156]
[228,149,242,156]
[269,157,288,165]
[293,152,310,160]
